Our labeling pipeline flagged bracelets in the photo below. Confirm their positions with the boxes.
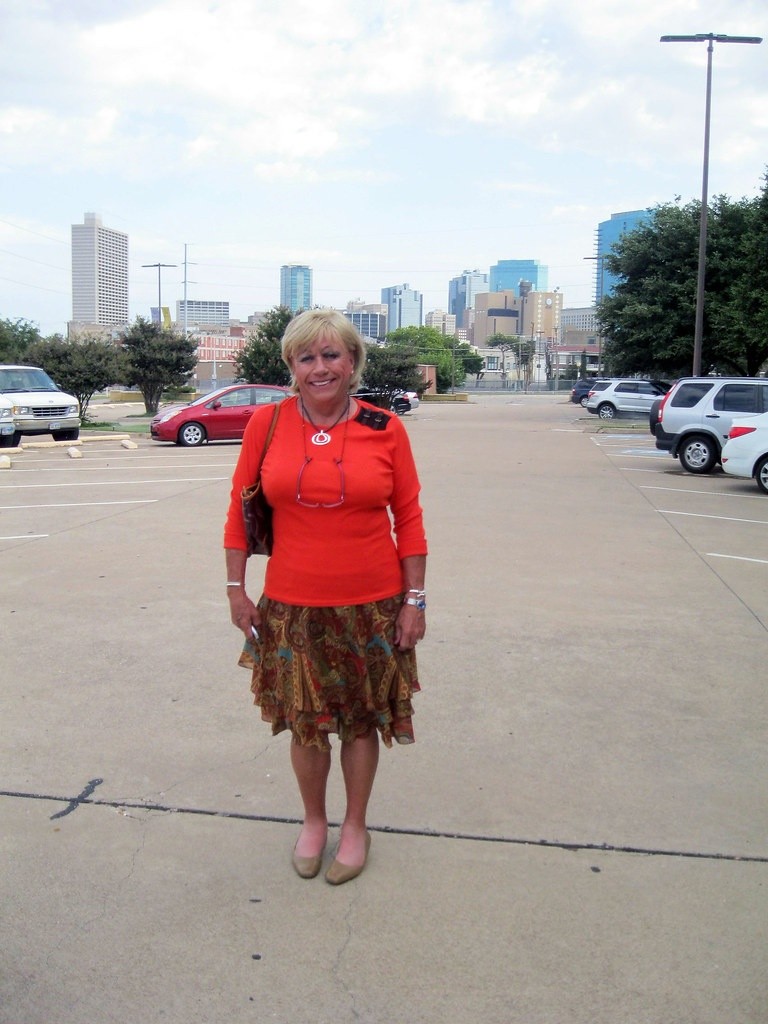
[408,589,426,596]
[227,580,246,588]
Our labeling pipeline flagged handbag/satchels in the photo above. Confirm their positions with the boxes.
[241,401,280,556]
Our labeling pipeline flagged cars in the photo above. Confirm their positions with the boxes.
[350,386,411,414]
[150,385,296,447]
[398,389,419,415]
[721,411,768,495]
[0,393,16,448]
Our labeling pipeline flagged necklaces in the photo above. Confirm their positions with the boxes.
[301,393,349,446]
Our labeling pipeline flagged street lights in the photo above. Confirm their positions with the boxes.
[659,33,763,378]
[141,263,178,331]
[584,255,622,377]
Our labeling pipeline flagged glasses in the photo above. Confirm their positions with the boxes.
[296,458,345,509]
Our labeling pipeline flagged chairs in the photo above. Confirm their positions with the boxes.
[237,391,249,404]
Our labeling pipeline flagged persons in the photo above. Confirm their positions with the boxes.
[223,308,427,885]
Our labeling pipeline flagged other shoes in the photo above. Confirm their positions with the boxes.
[293,824,327,878]
[326,829,372,885]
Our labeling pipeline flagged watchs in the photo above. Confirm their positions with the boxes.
[403,598,426,610]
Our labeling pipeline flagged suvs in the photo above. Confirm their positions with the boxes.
[587,381,666,420]
[0,365,81,447]
[571,377,610,408]
[649,377,768,474]
